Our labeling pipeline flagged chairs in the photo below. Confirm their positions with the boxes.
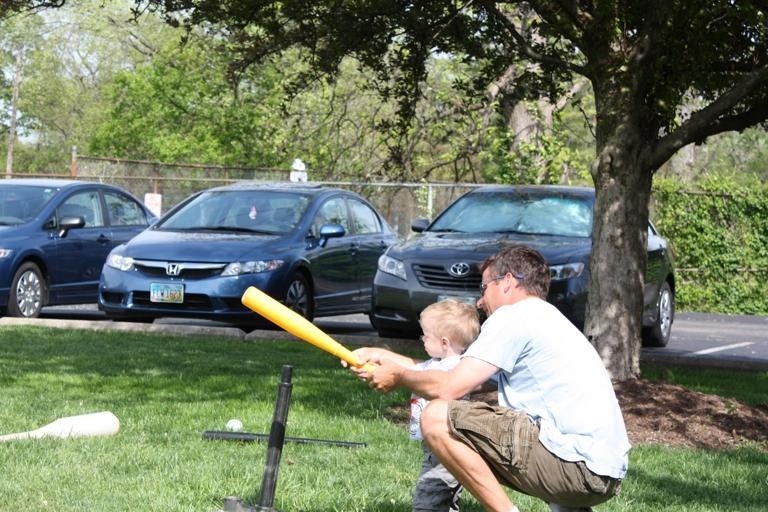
[231,203,296,230]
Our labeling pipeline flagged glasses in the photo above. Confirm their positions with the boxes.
[478,274,525,295]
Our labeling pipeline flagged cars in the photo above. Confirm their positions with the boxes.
[0,179,160,318]
[97,184,407,332]
[372,185,675,348]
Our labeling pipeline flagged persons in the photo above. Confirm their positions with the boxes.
[407,296,498,510]
[342,240,631,511]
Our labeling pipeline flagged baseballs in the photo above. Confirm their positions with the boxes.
[226,419,243,432]
